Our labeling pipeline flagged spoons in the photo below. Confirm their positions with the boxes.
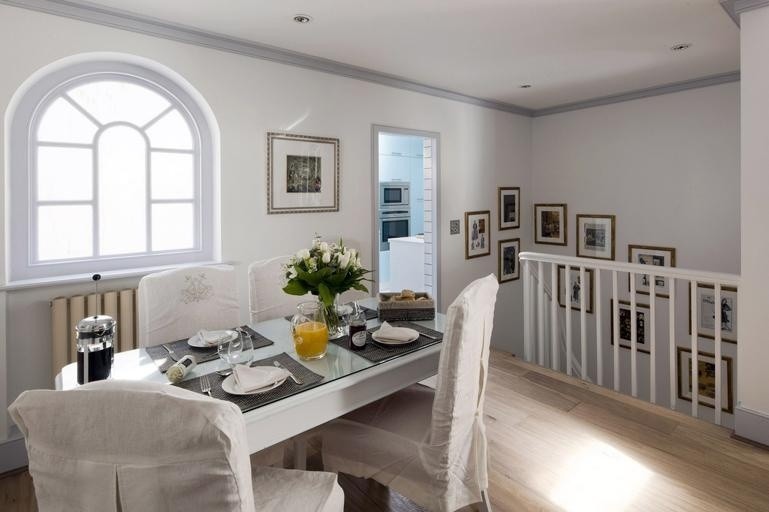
[214,367,253,376]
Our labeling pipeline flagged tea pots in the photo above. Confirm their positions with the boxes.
[290,301,328,360]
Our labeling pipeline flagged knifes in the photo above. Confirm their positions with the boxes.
[162,343,179,362]
[397,326,440,341]
[273,359,303,384]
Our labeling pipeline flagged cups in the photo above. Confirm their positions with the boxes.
[229,336,254,367]
[217,332,242,364]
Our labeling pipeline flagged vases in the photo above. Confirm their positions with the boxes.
[314,300,338,336]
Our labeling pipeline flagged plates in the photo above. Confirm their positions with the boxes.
[221,366,288,394]
[335,305,353,314]
[187,329,238,347]
[371,327,419,344]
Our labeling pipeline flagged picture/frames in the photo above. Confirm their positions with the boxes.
[497,186,520,231]
[556,264,595,315]
[628,245,676,298]
[676,346,733,415]
[498,238,520,283]
[688,281,737,344]
[534,203,567,246]
[465,209,491,259]
[575,214,615,260]
[610,298,650,355]
[266,131,339,214]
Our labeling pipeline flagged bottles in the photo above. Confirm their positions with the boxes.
[348,299,367,350]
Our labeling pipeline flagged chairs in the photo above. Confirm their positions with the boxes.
[5,376,344,512]
[320,272,500,512]
[248,255,317,324]
[136,263,239,346]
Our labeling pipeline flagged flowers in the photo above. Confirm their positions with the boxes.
[278,231,377,330]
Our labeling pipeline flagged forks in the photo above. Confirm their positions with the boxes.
[200,375,213,401]
[236,327,255,339]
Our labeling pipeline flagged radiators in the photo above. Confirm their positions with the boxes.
[51,288,138,375]
